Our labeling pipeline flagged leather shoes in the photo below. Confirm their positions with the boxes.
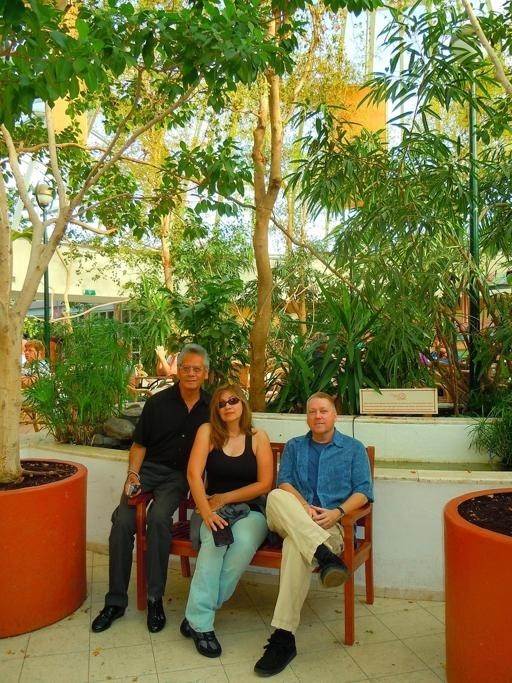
[318,551,348,588]
[91,603,125,633]
[147,597,166,633]
[254,627,297,677]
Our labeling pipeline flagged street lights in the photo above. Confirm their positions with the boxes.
[449,20,486,405]
[31,183,55,366]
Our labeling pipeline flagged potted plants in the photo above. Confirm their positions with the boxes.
[0,1,298,640]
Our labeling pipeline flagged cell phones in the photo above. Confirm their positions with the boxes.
[212,518,234,547]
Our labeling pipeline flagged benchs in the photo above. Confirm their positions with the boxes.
[128,436,377,648]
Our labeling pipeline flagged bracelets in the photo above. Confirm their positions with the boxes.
[303,502,311,507]
[335,505,346,517]
[126,468,140,479]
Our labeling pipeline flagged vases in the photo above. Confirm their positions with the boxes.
[441,488,512,683]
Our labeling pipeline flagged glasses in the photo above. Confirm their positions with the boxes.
[218,397,240,408]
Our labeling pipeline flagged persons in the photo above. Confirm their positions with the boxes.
[180,380,276,659]
[90,341,216,634]
[19,338,53,390]
[252,389,376,675]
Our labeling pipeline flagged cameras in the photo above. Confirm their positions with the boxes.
[127,484,140,496]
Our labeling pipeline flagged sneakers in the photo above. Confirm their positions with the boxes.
[180,614,222,658]
